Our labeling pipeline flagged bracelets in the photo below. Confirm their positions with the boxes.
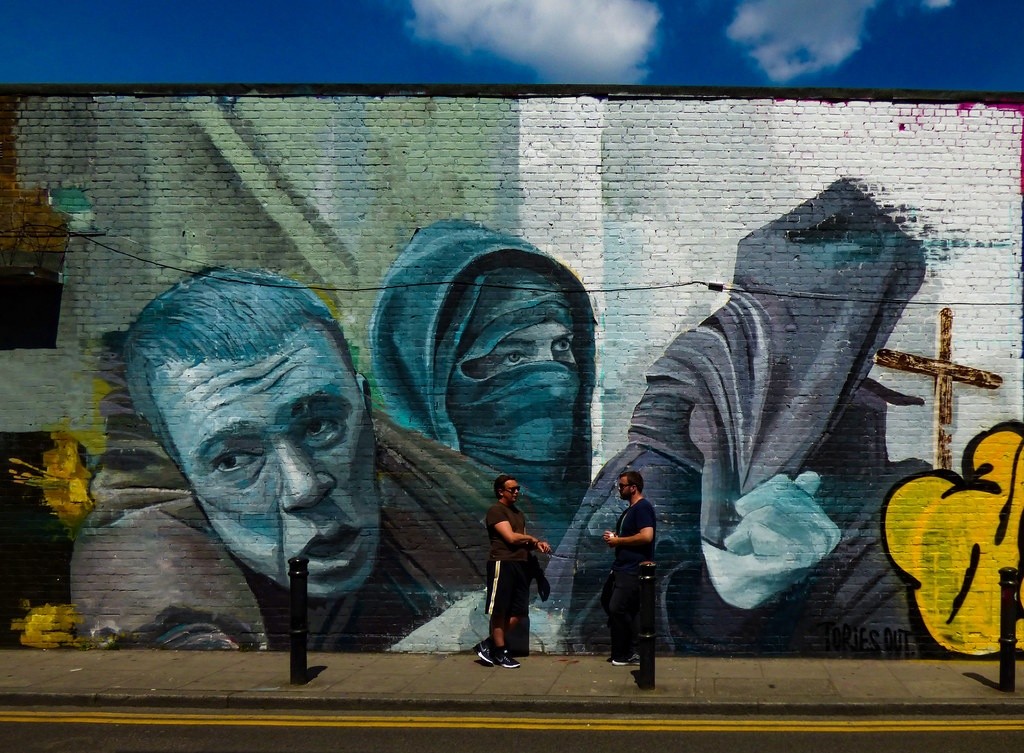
[536,541,540,547]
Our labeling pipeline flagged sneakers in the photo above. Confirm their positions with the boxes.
[472,640,494,665]
[494,649,520,668]
[612,653,641,666]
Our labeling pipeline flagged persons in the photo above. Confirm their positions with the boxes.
[473,475,553,668]
[598,471,656,667]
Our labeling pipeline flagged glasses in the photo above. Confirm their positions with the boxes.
[500,486,521,493]
[619,483,635,488]
